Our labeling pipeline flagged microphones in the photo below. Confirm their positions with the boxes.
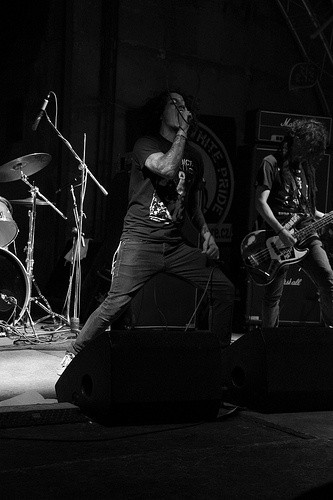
[56,175,81,194]
[187,116,193,123]
[32,94,50,131]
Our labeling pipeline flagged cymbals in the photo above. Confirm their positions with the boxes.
[1,152,52,183]
[10,197,55,206]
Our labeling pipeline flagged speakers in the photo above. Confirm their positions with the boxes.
[232,145,333,334]
[55,326,332,427]
[113,271,198,329]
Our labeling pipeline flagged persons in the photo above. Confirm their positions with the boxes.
[56,87,239,419]
[251,116,333,327]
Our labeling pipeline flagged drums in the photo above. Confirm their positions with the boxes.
[0,196,19,249]
[0,247,32,334]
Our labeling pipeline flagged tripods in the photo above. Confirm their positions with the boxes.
[5,110,108,338]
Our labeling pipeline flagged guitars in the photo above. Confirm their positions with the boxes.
[239,210,333,287]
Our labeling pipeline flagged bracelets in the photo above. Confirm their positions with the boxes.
[275,227,285,236]
[175,134,187,140]
